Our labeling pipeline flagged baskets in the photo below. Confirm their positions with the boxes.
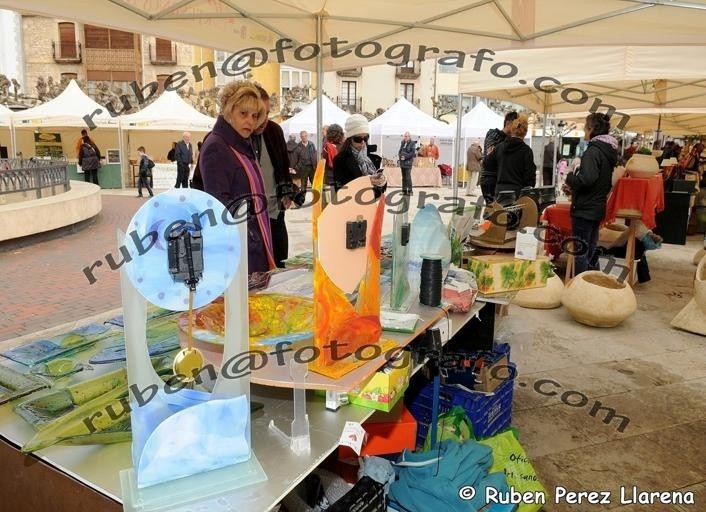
[405,359,514,448]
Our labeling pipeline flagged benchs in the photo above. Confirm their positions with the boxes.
[564,209,643,288]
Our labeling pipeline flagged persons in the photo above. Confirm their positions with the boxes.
[166,132,204,188]
[286,113,387,198]
[136,145,154,197]
[566,113,620,275]
[246,84,292,268]
[465,112,535,205]
[543,136,560,185]
[624,137,683,172]
[78,136,100,186]
[398,132,438,197]
[189,82,278,274]
[76,130,94,159]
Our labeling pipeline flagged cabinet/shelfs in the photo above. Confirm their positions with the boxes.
[0,268,495,512]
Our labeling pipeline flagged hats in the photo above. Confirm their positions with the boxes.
[345,114,370,139]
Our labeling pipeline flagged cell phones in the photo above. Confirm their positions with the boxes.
[376,168,384,173]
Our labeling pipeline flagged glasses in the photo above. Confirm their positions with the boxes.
[353,136,369,143]
